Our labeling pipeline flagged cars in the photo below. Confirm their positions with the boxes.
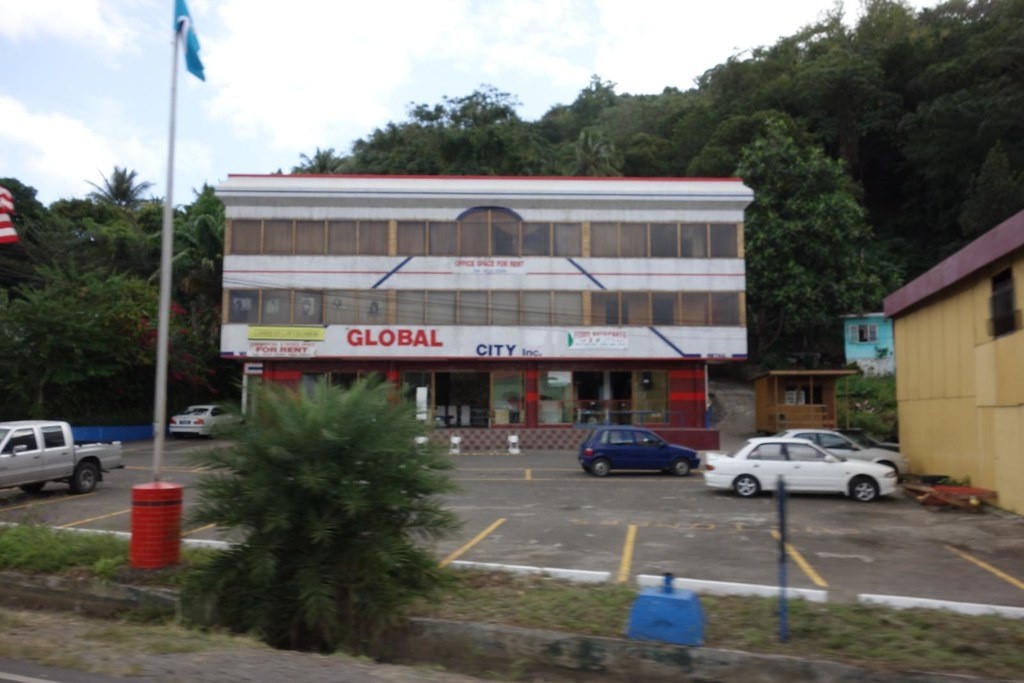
[701,436,899,505]
[578,426,701,478]
[773,428,906,478]
[170,402,243,439]
[832,429,903,450]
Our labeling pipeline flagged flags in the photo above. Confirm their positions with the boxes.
[173,0,206,83]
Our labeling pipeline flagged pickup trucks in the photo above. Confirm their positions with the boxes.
[0,418,126,495]
